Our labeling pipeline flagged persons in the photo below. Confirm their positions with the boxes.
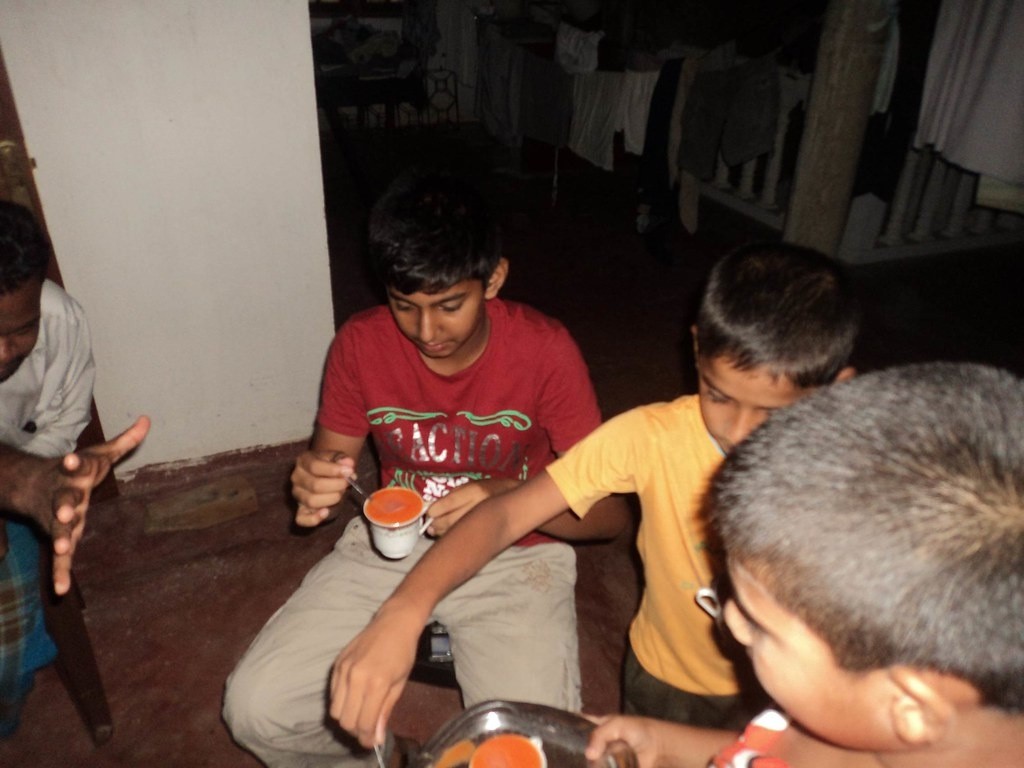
[324,241,859,768]
[579,359,1023,767]
[0,200,151,741]
[220,164,630,768]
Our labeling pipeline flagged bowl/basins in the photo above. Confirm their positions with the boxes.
[412,699,637,768]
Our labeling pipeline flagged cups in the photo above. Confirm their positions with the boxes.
[363,488,434,560]
